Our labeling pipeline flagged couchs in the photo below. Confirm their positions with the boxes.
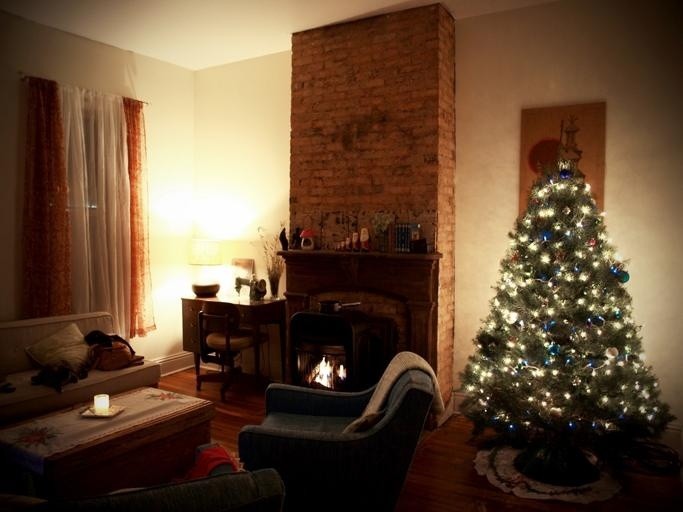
[0,312,160,420]
[0,468,286,512]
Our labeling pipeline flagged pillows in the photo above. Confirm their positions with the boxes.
[25,323,96,372]
[341,410,385,432]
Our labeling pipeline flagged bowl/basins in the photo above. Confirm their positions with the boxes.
[192,284,220,297]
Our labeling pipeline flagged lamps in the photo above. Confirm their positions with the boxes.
[188,243,224,297]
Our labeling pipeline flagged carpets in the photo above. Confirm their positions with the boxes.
[472,446,624,505]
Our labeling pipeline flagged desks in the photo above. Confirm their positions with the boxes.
[182,296,288,384]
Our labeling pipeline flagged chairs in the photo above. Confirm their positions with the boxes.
[196,311,256,402]
[238,350,444,512]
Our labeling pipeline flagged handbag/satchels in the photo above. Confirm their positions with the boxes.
[93,341,132,370]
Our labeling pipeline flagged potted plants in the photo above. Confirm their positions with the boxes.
[257,216,287,298]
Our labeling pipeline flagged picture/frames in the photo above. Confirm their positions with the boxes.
[519,101,606,216]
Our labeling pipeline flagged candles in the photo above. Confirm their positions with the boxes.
[94,393,109,410]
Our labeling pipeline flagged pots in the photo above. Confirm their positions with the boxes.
[319,301,361,314]
